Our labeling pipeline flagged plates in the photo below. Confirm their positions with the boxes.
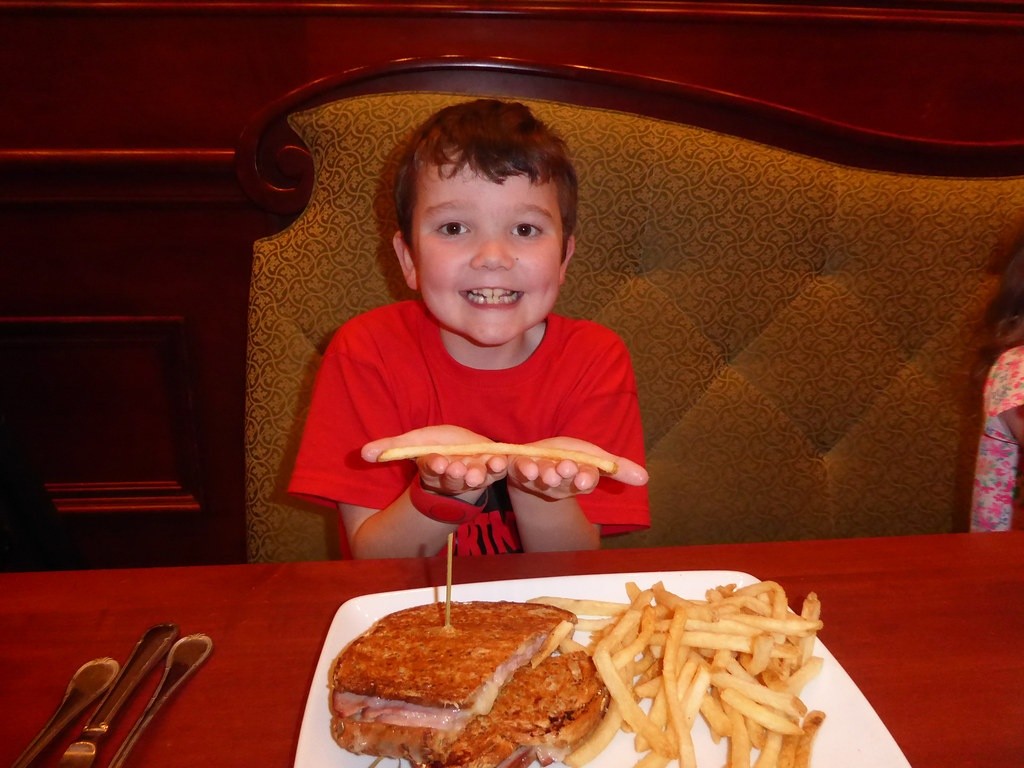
[293,570,913,768]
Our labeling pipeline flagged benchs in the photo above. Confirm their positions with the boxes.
[234,54,1023,562]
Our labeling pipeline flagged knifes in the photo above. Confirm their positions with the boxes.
[56,622,179,768]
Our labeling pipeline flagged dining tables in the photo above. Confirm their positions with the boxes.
[0,527,1023,768]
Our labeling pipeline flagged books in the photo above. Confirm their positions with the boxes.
[44,480,202,512]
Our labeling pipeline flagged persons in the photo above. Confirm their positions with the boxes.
[965,225,1024,533]
[291,100,652,560]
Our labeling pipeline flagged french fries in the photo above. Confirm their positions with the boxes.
[377,443,619,474]
[524,580,827,768]
